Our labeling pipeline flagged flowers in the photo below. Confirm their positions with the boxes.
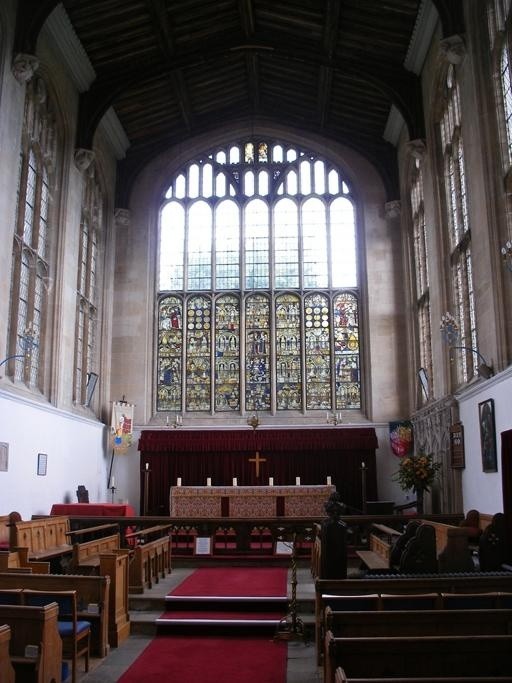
[389,446,443,496]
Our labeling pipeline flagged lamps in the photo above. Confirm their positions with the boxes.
[439,311,494,379]
[0,320,38,367]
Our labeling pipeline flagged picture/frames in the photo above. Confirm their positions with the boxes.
[38,453,48,476]
[417,368,429,400]
[477,397,498,474]
[86,372,99,407]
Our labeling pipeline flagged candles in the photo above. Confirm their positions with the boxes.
[180,416,182,423]
[176,415,178,421]
[326,412,328,419]
[146,462,149,469]
[362,461,365,468]
[336,413,338,420]
[340,412,341,418]
[167,415,168,423]
[111,476,115,486]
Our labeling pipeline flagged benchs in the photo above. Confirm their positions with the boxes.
[311,510,511,683]
[1,512,172,683]
